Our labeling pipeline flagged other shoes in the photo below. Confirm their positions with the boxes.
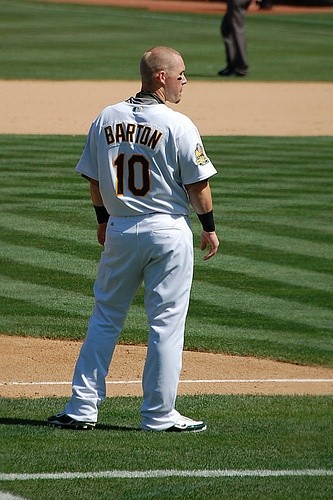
[218,68,247,77]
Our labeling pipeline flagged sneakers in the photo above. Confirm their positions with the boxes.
[48,413,96,429]
[143,416,206,433]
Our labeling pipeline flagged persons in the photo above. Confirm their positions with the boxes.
[48,46,219,433]
[218,0,252,76]
